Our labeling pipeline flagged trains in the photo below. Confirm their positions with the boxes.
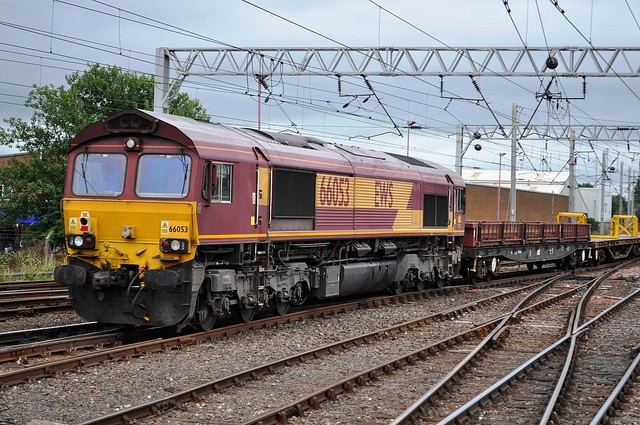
[53,107,629,333]
